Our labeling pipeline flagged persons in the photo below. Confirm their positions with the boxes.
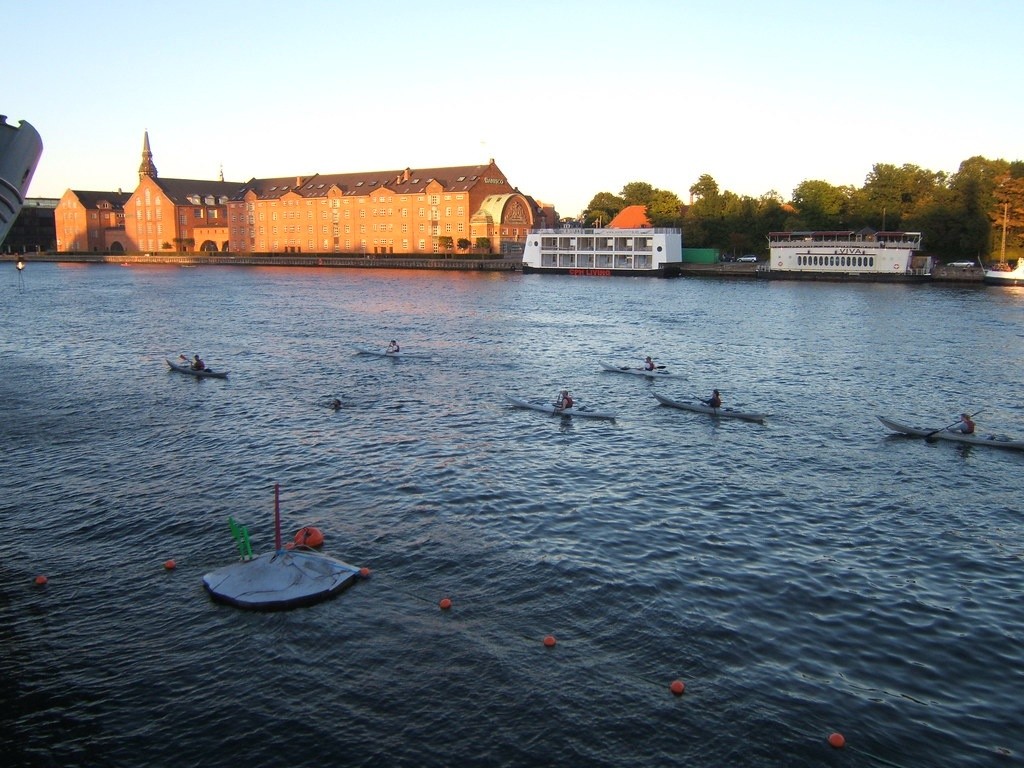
[555,389,573,411]
[948,412,974,434]
[333,399,343,409]
[703,389,721,408]
[643,356,656,371]
[190,355,205,370]
[386,339,400,353]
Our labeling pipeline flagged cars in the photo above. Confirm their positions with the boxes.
[946,259,975,268]
[721,254,732,262]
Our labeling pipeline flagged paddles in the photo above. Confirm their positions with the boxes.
[180,354,195,364]
[694,396,709,404]
[620,366,666,370]
[550,393,561,418]
[926,409,985,438]
[379,343,391,358]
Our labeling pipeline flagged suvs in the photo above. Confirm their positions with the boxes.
[737,254,759,263]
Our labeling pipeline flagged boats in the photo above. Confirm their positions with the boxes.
[166,360,232,378]
[596,359,691,380]
[756,230,934,282]
[357,346,432,359]
[651,390,767,421]
[983,270,1024,286]
[505,394,622,419]
[876,415,1024,450]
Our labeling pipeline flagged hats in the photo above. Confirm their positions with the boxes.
[960,413,971,420]
[560,391,568,394]
[646,356,652,361]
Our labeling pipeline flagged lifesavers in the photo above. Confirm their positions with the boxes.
[318,259,324,265]
[294,526,324,547]
[511,266,515,271]
[912,259,917,268]
[479,264,483,269]
